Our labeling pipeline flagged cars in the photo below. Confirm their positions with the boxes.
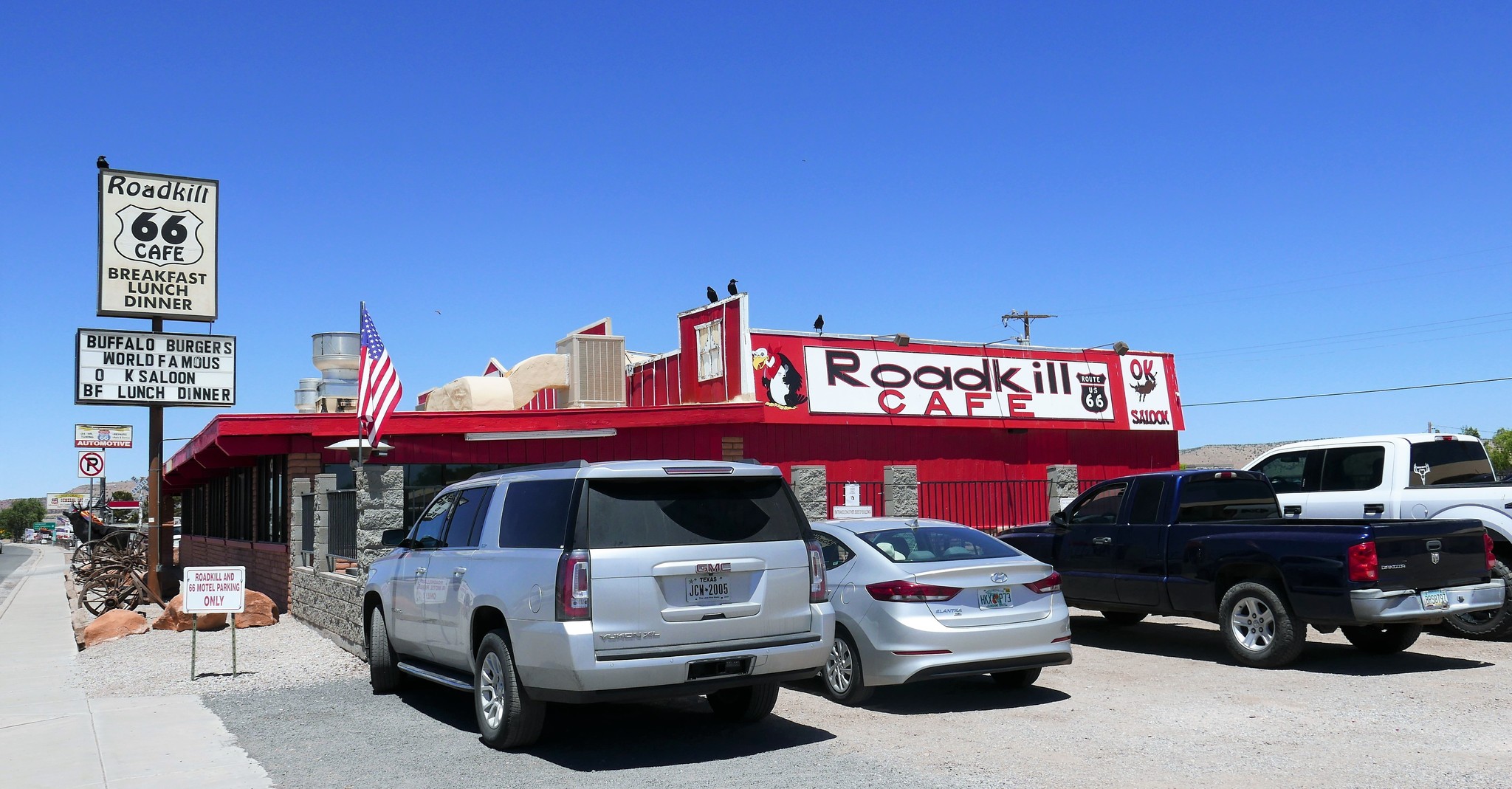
[810,518,1073,706]
[0,537,4,554]
[66,536,80,547]
[173,524,181,548]
[136,530,149,544]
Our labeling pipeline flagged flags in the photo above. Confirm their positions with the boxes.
[357,302,402,448]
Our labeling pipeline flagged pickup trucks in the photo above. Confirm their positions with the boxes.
[1241,433,1512,640]
[997,469,1505,669]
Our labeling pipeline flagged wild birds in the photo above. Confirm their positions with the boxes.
[728,279,739,296]
[813,314,824,333]
[707,287,718,303]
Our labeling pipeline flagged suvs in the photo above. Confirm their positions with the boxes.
[362,458,837,749]
